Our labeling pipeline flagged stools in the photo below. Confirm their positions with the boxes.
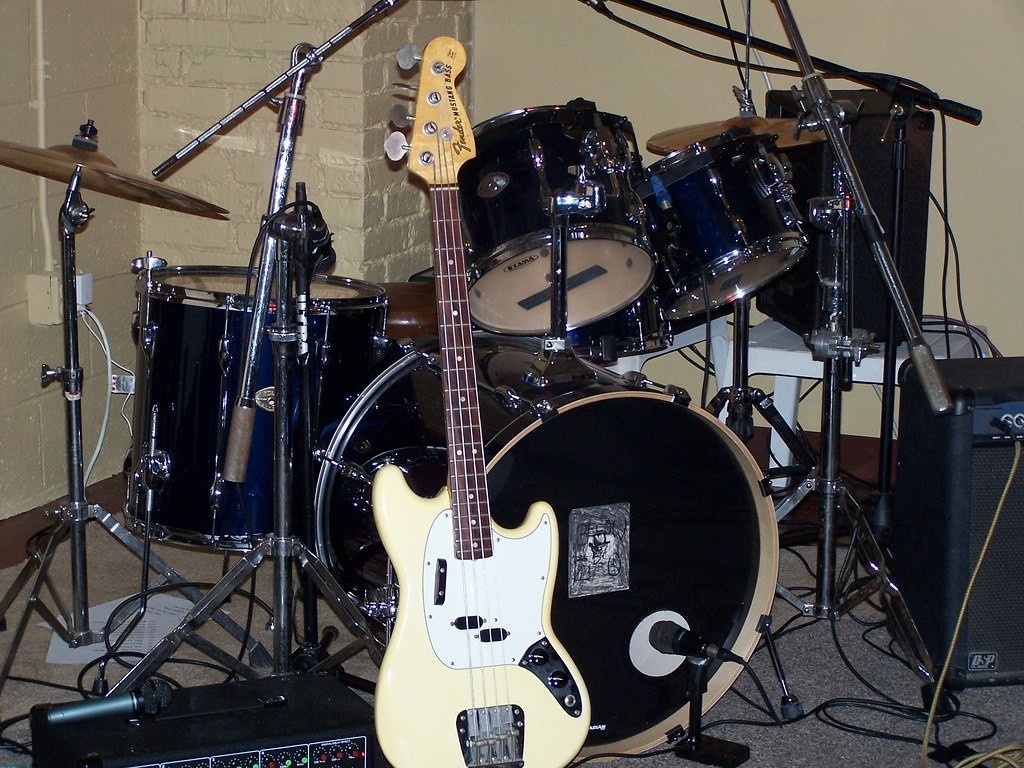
[719,318,988,492]
[603,319,728,412]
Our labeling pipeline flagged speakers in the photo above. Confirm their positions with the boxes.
[897,356,1024,690]
[763,88,936,346]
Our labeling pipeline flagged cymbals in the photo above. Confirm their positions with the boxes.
[0,139,234,222]
[646,115,830,156]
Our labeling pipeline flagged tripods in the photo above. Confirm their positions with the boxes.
[0,0,399,698]
[610,0,961,716]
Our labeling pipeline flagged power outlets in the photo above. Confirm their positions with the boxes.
[26,271,81,325]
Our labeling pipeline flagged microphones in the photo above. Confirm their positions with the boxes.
[649,620,745,665]
[310,205,336,270]
[47,679,175,725]
[585,0,613,18]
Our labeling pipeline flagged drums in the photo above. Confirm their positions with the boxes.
[307,324,782,763]
[455,98,660,337]
[124,262,389,554]
[562,126,813,363]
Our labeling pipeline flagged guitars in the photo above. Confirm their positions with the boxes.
[370,33,594,767]
